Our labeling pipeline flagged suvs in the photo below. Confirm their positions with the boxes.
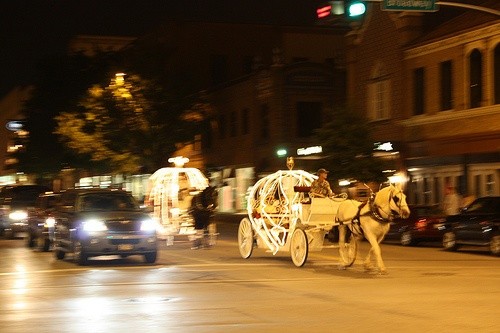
[49,184,160,267]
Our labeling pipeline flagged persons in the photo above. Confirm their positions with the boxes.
[310,168,335,198]
[443,186,460,216]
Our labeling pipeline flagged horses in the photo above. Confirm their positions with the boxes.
[190,183,219,250]
[334,181,411,276]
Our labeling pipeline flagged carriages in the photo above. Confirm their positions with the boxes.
[140,156,227,251]
[237,155,411,276]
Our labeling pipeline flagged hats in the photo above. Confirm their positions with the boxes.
[318,168,329,174]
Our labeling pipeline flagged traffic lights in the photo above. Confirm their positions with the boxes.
[310,0,370,24]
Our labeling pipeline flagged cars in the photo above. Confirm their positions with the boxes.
[442,195,500,255]
[28,191,64,247]
[0,185,53,238]
[328,195,450,248]
